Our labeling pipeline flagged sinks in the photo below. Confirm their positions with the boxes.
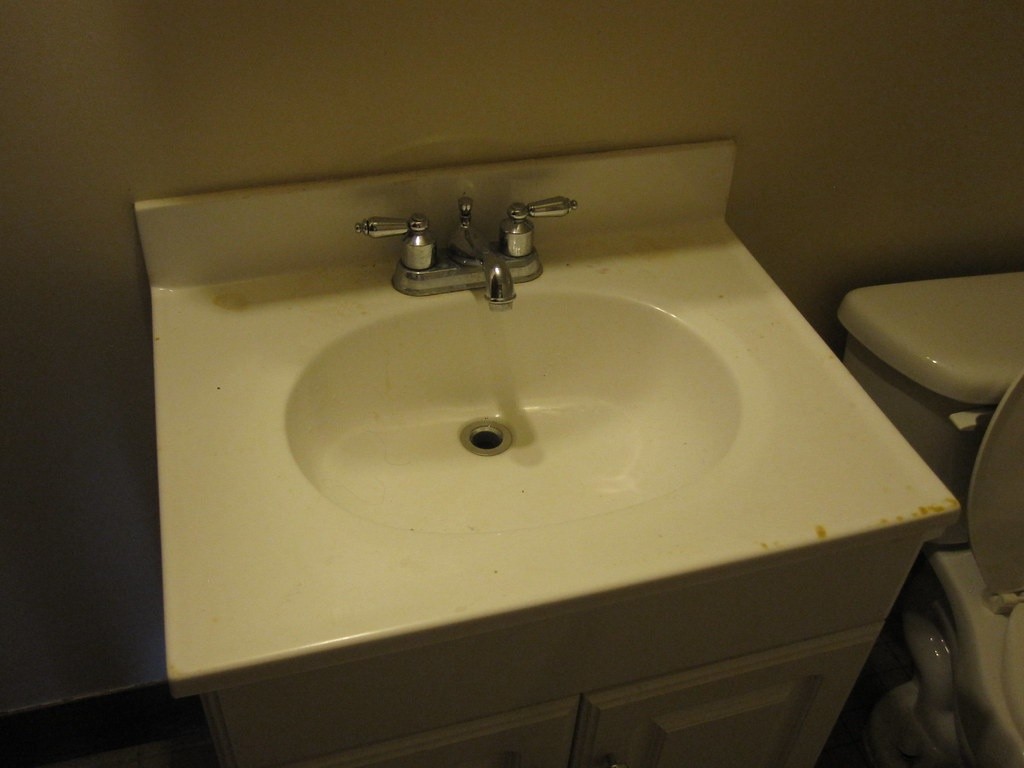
[280,288,744,536]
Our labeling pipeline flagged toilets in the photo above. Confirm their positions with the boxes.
[836,264,1024,768]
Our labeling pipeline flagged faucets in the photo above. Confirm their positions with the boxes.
[355,195,576,312]
